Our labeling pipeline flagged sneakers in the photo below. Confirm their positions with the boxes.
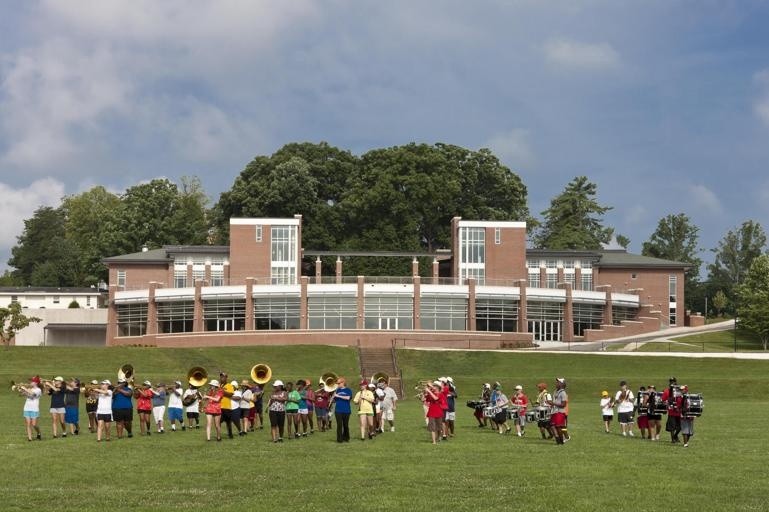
[272,425,333,442]
[217,425,264,440]
[442,434,447,440]
[368,426,395,438]
[53,423,199,441]
[480,423,570,444]
[37,432,41,440]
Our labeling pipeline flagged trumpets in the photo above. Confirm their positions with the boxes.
[414,381,429,398]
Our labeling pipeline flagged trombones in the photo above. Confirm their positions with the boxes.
[10,376,104,395]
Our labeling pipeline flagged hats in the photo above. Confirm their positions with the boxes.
[220,373,228,378]
[272,379,284,387]
[91,379,128,385]
[556,377,566,387]
[71,376,80,385]
[29,376,40,383]
[144,380,181,387]
[536,383,547,388]
[359,376,387,388]
[514,385,523,391]
[54,375,64,382]
[231,379,238,389]
[432,376,454,388]
[208,379,220,387]
[337,378,345,384]
[239,379,252,388]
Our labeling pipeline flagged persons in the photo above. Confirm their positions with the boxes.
[352,377,398,440]
[600,377,693,447]
[83,371,133,442]
[149,383,166,433]
[328,377,353,443]
[45,376,68,438]
[474,377,571,446]
[265,379,333,443]
[17,376,44,442]
[134,380,154,436]
[183,383,203,429]
[64,377,81,435]
[202,372,266,442]
[165,380,186,431]
[419,377,457,445]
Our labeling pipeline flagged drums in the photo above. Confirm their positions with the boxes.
[638,385,704,419]
[466,400,498,418]
[525,406,552,423]
[508,408,520,419]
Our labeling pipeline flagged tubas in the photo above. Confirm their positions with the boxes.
[319,372,339,393]
[181,366,207,406]
[371,372,390,387]
[118,365,150,395]
[251,364,271,398]
